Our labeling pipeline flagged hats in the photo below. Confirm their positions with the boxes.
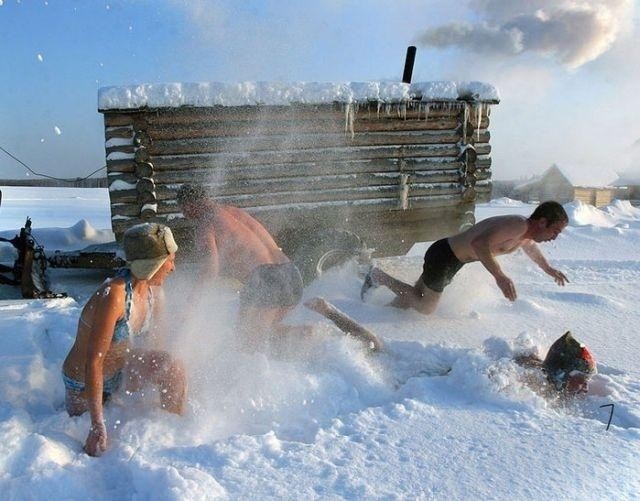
[542,331,597,391]
[124,222,179,281]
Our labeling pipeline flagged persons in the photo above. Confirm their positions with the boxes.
[176,180,316,358]
[62,223,189,458]
[302,296,598,407]
[360,200,569,315]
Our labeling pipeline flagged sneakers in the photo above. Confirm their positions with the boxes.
[361,265,378,302]
